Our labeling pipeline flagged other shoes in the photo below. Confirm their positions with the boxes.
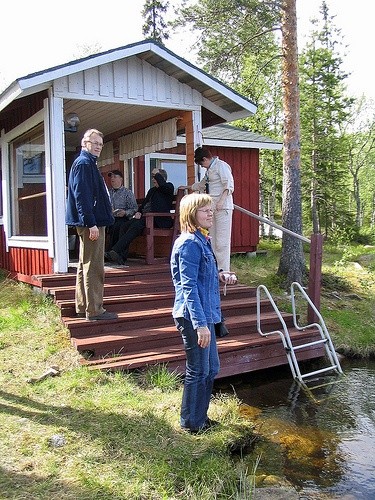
[188,429,210,435]
[109,251,126,265]
[76,311,86,317]
[86,310,118,320]
[104,251,112,262]
[205,418,221,426]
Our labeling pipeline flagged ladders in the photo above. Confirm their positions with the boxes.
[257,282,342,381]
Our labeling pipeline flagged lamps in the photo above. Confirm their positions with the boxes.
[65,113,81,132]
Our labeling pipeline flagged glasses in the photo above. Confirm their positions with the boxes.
[87,140,105,147]
[197,208,214,214]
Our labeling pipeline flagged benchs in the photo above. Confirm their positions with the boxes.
[136,189,185,265]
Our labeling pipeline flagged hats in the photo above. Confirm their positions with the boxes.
[108,170,123,178]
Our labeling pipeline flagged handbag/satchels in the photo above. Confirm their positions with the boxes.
[214,312,229,338]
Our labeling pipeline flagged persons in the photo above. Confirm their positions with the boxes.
[177,146,234,284]
[104,168,174,265]
[170,193,237,437]
[105,169,138,260]
[66,128,120,321]
